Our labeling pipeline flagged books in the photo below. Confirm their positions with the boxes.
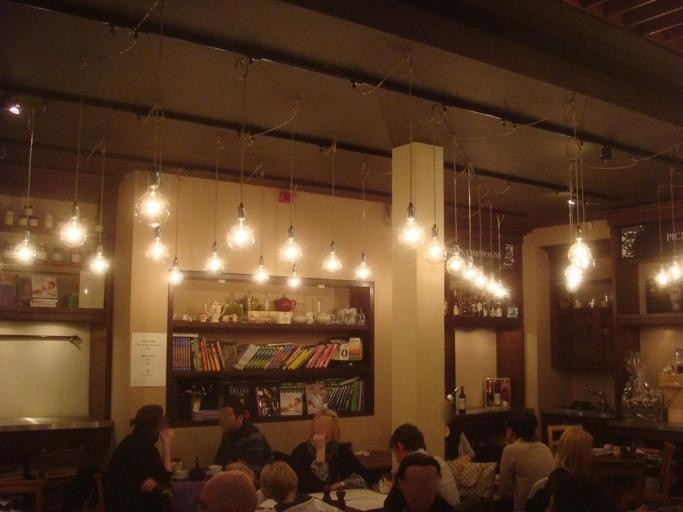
[46,459,75,478]
[169,333,364,420]
[17,271,104,310]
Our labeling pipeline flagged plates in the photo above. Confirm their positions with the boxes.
[171,475,190,481]
[204,471,226,476]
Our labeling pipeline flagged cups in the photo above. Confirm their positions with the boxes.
[208,464,222,473]
[175,470,187,477]
[196,312,209,323]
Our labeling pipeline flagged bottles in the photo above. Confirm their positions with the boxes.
[3,202,85,268]
[457,386,467,415]
[560,291,609,308]
[451,289,503,317]
[446,387,458,400]
[486,380,502,406]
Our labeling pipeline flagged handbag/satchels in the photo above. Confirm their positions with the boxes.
[445,461,497,498]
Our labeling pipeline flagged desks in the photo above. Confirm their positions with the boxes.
[0,406,682,512]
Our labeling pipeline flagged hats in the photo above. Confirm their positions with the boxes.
[130,404,171,429]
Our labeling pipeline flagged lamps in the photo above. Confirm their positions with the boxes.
[250,163,269,286]
[204,155,224,277]
[563,115,595,294]
[89,146,112,276]
[14,104,43,267]
[356,158,373,278]
[324,141,342,272]
[0,406,682,512]
[656,169,682,287]
[167,175,183,285]
[145,227,169,265]
[134,147,170,227]
[9,101,24,115]
[227,142,255,251]
[61,100,92,249]
[280,130,303,262]
[399,100,506,297]
[287,262,303,287]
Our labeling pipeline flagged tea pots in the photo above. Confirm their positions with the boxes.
[293,313,308,324]
[305,312,318,325]
[271,295,295,311]
[316,313,336,325]
[204,298,226,323]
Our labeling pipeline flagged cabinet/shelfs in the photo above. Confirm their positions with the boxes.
[442,308,526,424]
[0,222,111,324]
[167,277,369,421]
[608,312,683,428]
[550,303,612,366]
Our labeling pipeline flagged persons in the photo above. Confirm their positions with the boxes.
[106,405,173,511]
[199,420,461,512]
[498,408,554,512]
[524,428,603,512]
